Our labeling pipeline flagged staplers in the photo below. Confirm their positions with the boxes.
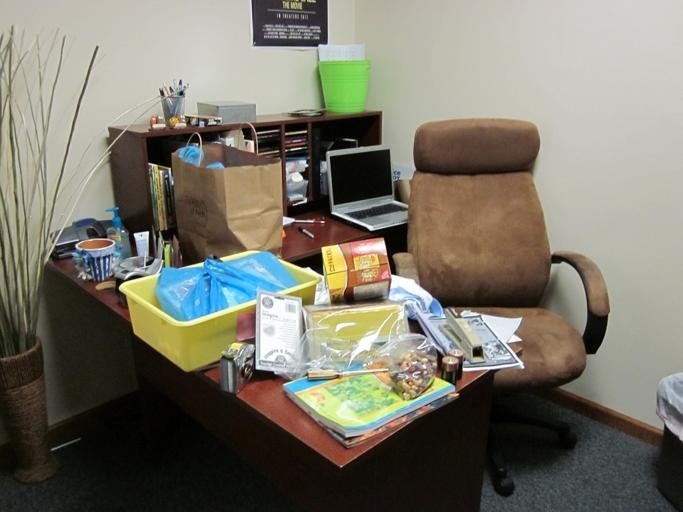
[438,306,486,364]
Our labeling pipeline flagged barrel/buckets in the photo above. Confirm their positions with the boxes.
[319,58,372,112]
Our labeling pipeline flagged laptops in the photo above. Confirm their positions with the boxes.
[325,145,409,232]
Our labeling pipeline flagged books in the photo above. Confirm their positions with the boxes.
[411,310,528,373]
[280,360,455,450]
[219,127,310,160]
[148,160,177,233]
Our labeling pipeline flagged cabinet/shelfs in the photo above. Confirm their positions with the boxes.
[106,107,383,239]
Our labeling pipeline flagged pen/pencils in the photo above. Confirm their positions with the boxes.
[299,226,315,238]
[159,79,189,124]
[295,220,325,224]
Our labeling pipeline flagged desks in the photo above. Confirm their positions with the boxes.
[43,192,524,511]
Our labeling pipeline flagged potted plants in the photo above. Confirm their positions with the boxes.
[0,23,187,486]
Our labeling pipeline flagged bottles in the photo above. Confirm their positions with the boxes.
[441,348,464,386]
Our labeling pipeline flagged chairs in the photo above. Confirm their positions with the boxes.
[391,116,610,498]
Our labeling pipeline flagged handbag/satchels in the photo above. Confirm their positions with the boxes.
[171,120,285,259]
